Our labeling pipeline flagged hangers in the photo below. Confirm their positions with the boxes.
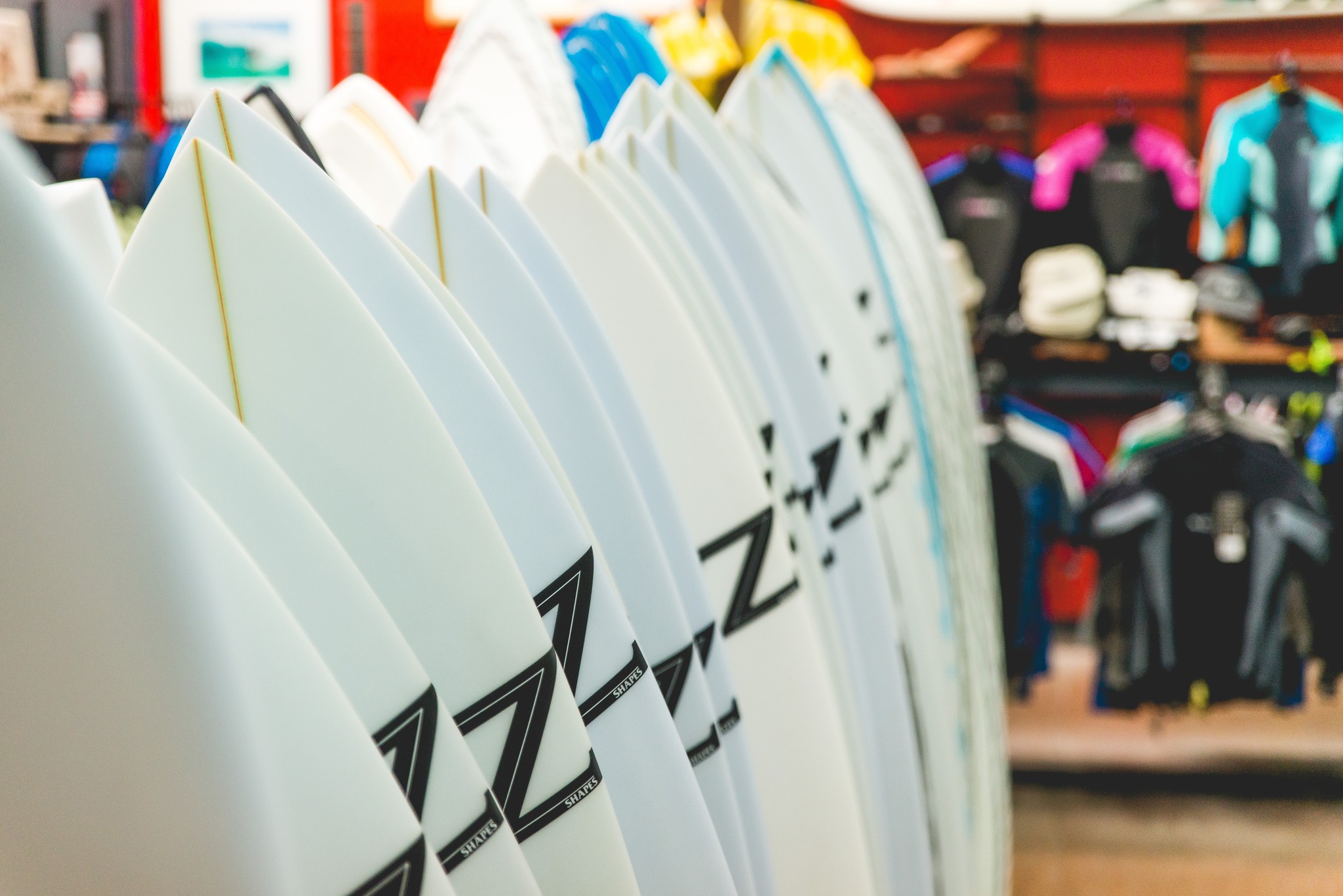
[923,65,1308,444]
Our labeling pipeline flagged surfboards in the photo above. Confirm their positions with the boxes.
[0,2,1016,896]
